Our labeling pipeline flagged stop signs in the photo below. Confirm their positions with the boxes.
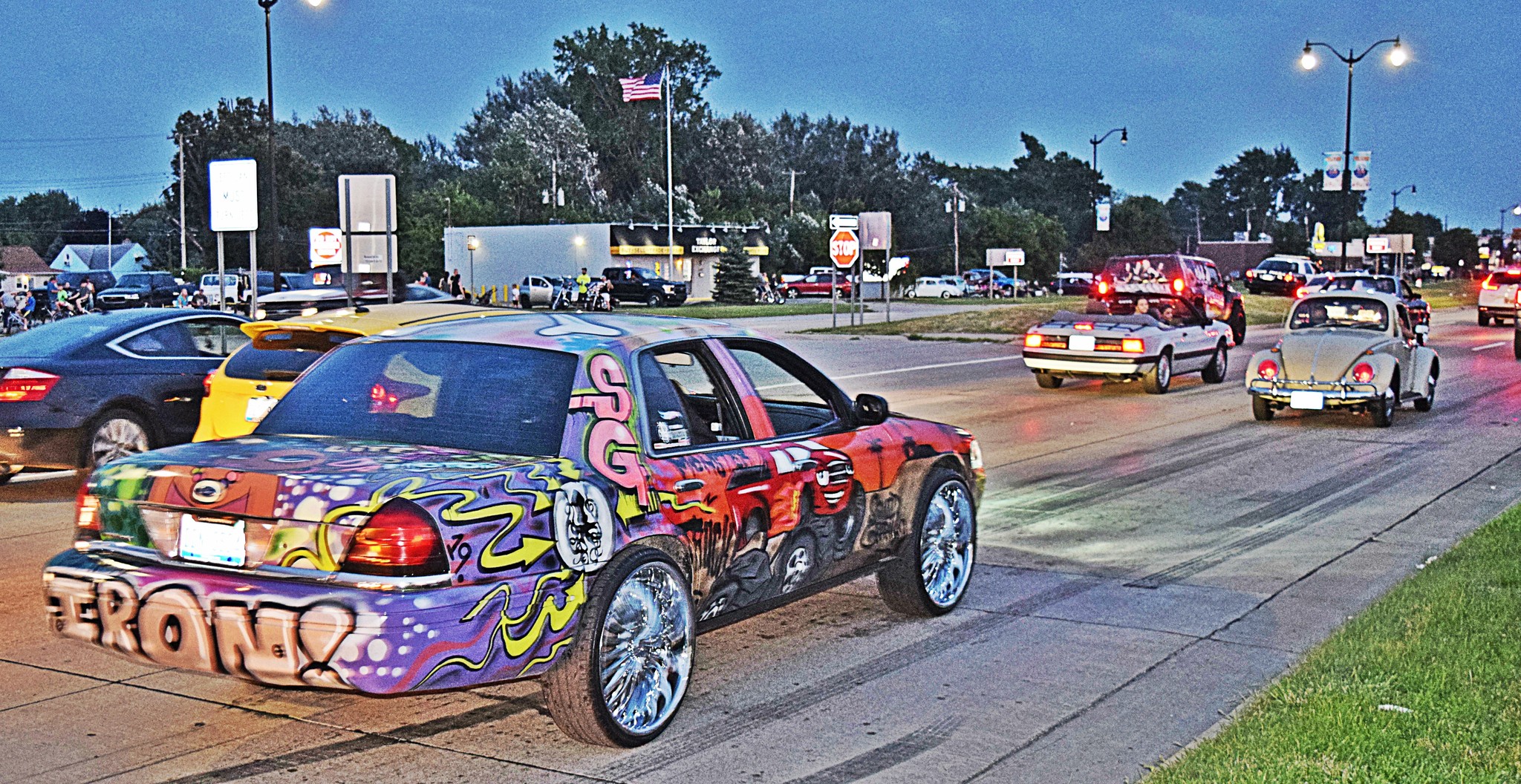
[830,230,859,268]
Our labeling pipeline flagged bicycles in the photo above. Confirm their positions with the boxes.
[0,298,103,335]
[752,281,786,305]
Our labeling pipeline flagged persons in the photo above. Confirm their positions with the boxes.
[757,272,770,302]
[576,267,591,310]
[0,291,17,334]
[21,291,36,330]
[176,289,195,309]
[597,275,614,313]
[415,269,467,299]
[511,283,519,308]
[192,289,211,310]
[44,276,96,317]
[1378,306,1417,338]
[1132,294,1150,315]
[1299,305,1327,328]
[1159,304,1173,324]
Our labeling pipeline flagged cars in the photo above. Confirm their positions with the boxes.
[190,303,538,445]
[42,310,985,749]
[1245,289,1441,428]
[0,307,256,488]
[1049,276,1093,297]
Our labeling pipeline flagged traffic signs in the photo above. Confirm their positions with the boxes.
[829,214,859,230]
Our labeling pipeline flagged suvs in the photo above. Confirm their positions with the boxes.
[1084,254,1520,360]
[776,274,858,300]
[96,267,459,323]
[27,269,116,319]
[601,267,687,308]
[519,274,603,309]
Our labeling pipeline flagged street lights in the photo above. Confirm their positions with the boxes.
[1500,202,1521,236]
[1088,125,1128,298]
[1390,184,1417,209]
[1301,32,1406,273]
[445,197,451,229]
[108,207,129,270]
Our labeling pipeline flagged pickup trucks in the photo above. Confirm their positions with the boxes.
[902,269,1028,300]
[780,267,843,284]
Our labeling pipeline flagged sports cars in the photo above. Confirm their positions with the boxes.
[1022,290,1235,394]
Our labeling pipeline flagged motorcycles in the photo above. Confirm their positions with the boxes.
[549,282,573,310]
